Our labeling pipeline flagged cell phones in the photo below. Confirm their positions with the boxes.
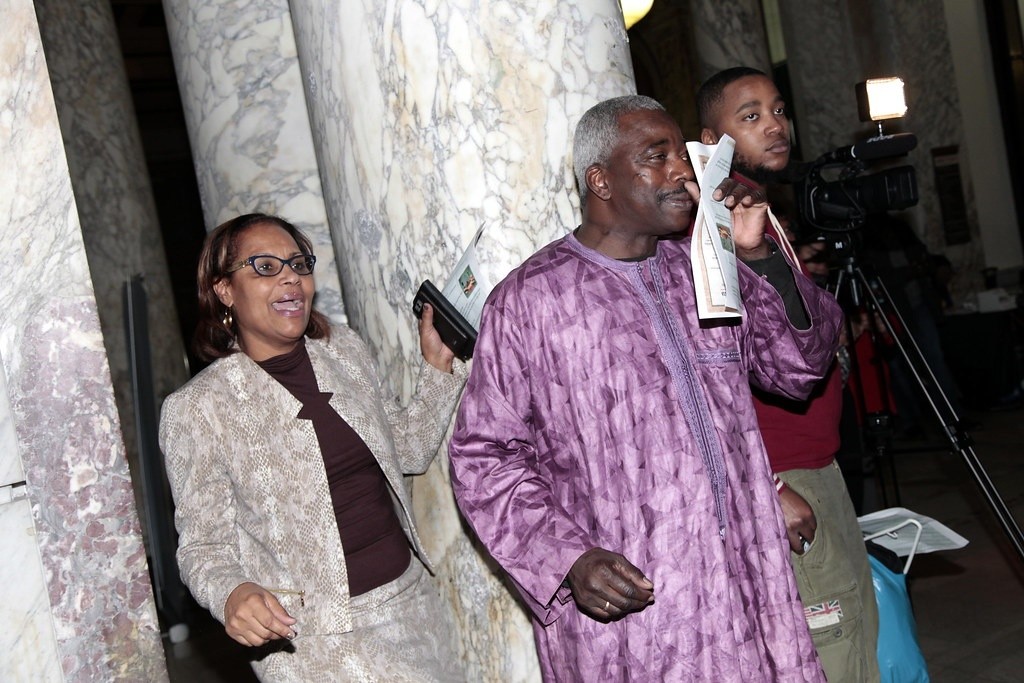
[412,291,468,357]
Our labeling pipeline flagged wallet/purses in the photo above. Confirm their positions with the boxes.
[414,279,479,363]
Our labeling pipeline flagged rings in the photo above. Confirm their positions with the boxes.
[603,602,609,610]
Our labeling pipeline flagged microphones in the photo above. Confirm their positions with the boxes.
[851,133,917,160]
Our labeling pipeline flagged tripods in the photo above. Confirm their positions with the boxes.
[820,234,1024,563]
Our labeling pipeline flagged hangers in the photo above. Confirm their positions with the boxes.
[862,518,922,577]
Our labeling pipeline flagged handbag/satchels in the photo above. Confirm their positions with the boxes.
[865,554,929,683]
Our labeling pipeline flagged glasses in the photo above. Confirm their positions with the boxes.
[224,255,316,277]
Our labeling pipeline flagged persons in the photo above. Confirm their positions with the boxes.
[686,65,898,683]
[446,94,844,683]
[159,213,469,683]
